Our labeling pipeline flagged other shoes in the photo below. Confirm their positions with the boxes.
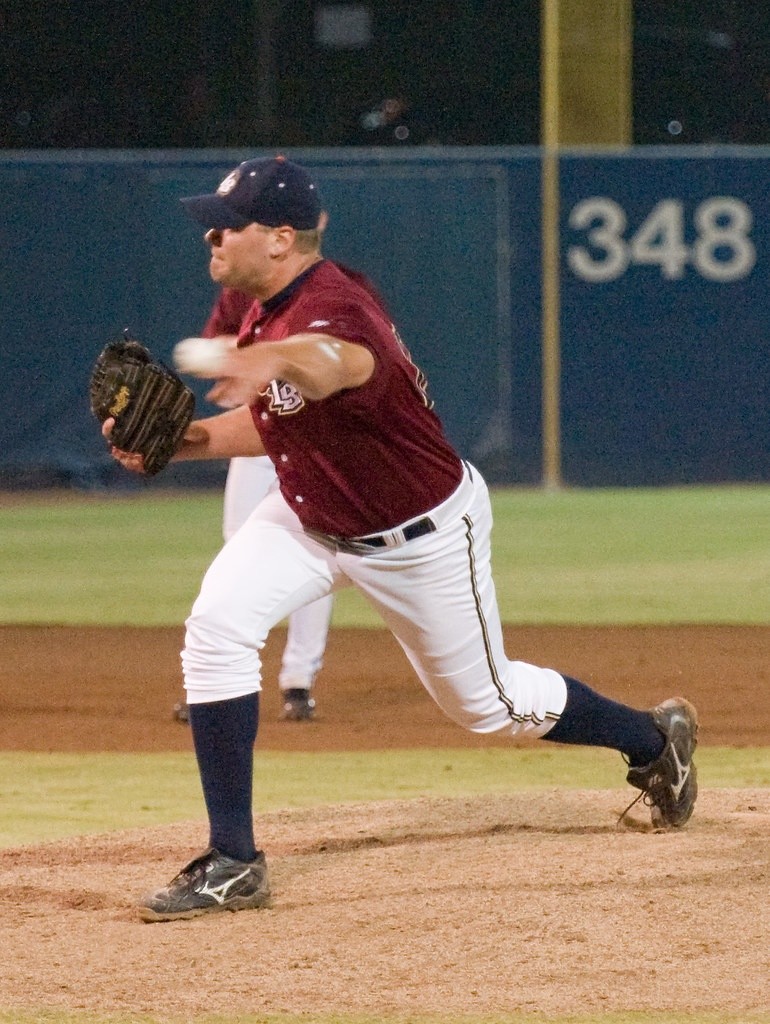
[172,698,191,724]
[278,692,314,722]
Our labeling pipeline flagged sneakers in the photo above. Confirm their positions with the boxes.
[135,845,271,922]
[616,695,699,830]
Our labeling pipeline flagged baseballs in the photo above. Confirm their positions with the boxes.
[173,335,221,371]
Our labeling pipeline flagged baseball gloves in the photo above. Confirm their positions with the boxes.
[89,337,201,479]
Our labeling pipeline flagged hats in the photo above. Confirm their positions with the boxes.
[181,155,322,229]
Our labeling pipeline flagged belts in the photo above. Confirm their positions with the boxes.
[340,459,472,553]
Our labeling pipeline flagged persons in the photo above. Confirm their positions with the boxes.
[0,66,540,145]
[100,155,701,922]
[172,211,333,724]
